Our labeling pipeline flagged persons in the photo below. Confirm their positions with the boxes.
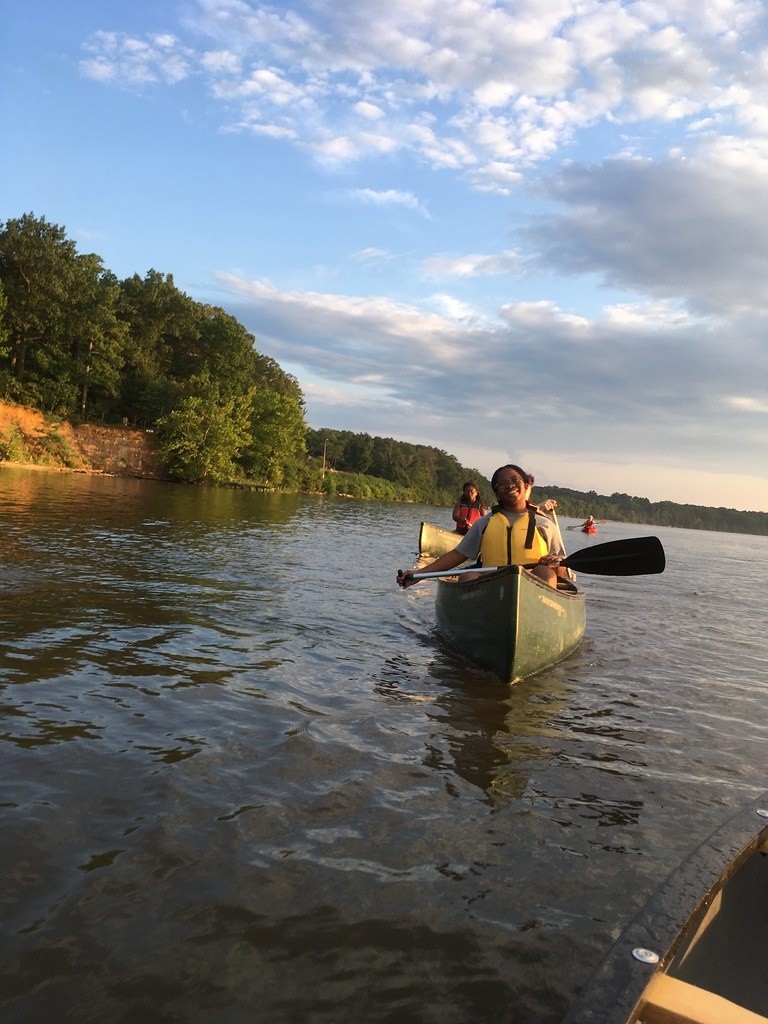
[395,464,567,590]
[580,515,596,529]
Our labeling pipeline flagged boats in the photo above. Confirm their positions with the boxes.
[418,521,467,560]
[436,571,588,688]
[622,807,768,1024]
[581,526,598,534]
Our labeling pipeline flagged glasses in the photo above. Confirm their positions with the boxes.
[494,476,522,489]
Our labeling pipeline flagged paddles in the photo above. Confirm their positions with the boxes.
[549,502,572,578]
[397,537,666,588]
[566,519,609,530]
